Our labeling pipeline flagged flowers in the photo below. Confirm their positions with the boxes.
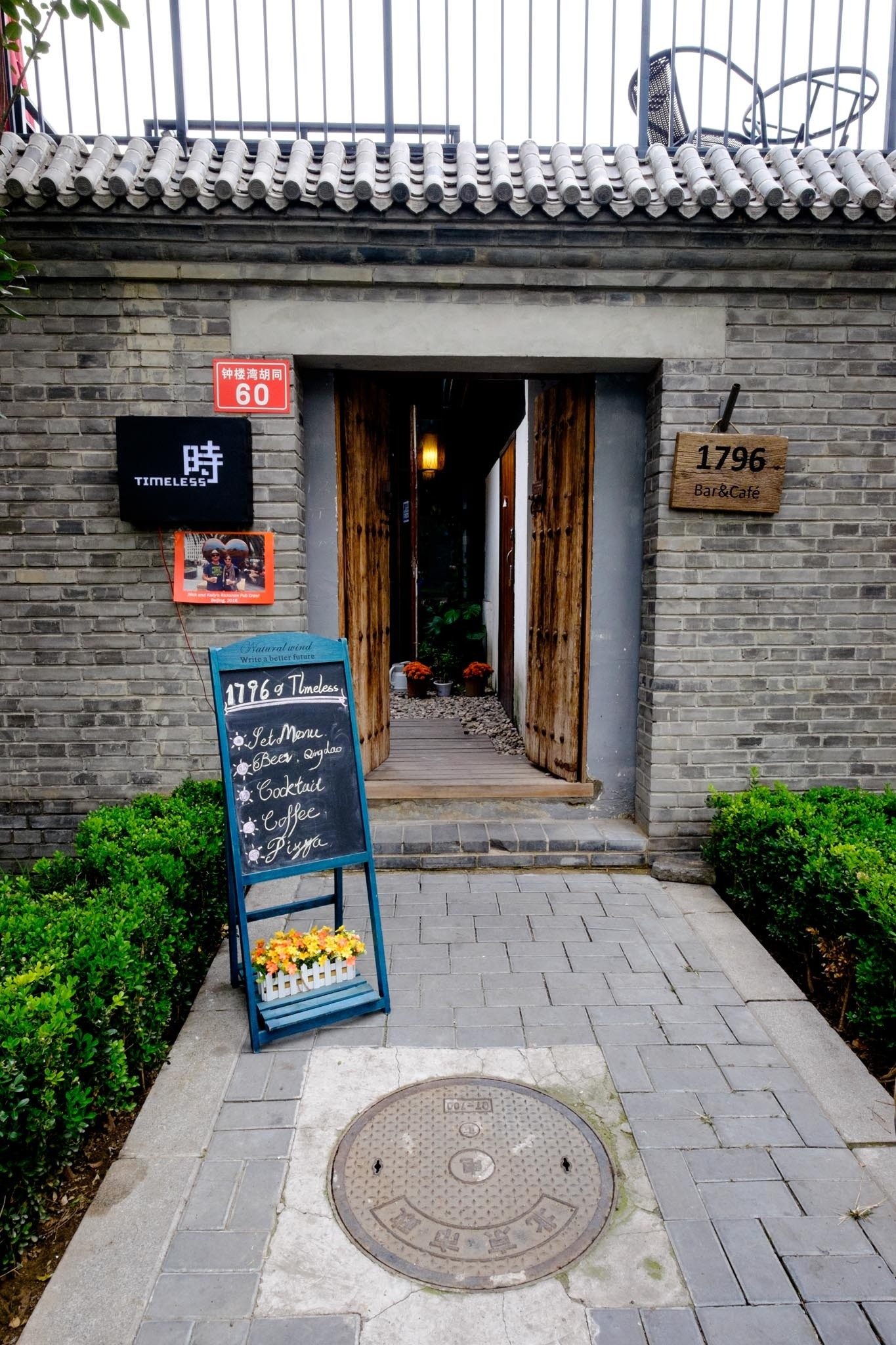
[251,925,364,998]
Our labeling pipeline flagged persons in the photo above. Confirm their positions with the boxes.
[238,562,247,574]
[249,562,262,584]
[202,557,210,568]
[259,567,265,577]
[257,561,262,572]
[196,557,199,565]
[202,549,226,591]
[221,554,241,592]
[244,561,252,583]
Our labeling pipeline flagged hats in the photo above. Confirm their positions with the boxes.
[210,549,220,557]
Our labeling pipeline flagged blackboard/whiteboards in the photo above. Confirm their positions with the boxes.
[210,634,375,886]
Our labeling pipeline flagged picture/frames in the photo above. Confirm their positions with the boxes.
[174,528,274,606]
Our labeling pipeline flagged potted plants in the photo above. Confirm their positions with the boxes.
[461,661,493,697]
[402,661,431,699]
[420,601,463,696]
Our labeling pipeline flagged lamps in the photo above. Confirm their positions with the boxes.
[416,431,445,478]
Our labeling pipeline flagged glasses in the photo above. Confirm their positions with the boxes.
[225,558,231,560]
[211,554,220,557]
